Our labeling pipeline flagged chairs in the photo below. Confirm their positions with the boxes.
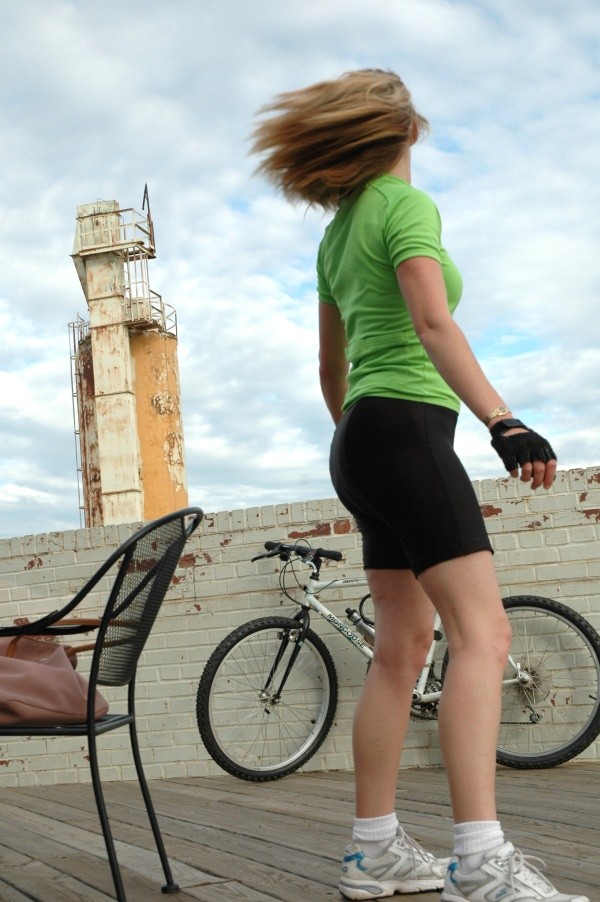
[0,509,206,902]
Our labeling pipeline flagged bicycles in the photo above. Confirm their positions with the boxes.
[193,534,600,782]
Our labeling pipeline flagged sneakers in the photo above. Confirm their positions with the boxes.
[337,825,453,900]
[442,840,589,902]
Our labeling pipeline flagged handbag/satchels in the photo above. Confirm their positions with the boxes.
[0,612,148,727]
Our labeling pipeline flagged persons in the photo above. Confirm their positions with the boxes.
[245,68,588,902]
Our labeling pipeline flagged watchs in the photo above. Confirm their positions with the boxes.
[484,407,512,426]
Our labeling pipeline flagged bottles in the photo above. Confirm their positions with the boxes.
[346,608,376,647]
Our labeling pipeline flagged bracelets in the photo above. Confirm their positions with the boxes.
[489,419,527,438]
